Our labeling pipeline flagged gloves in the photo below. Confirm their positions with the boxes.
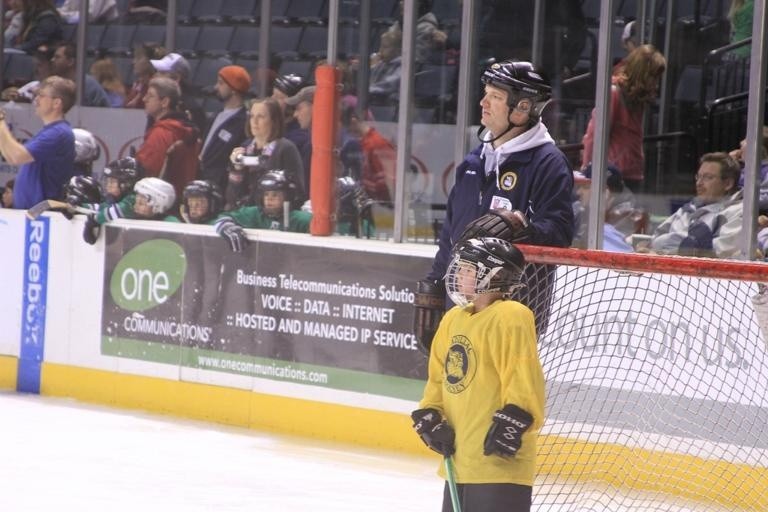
[337,177,368,214]
[223,224,247,252]
[484,404,533,456]
[412,408,456,454]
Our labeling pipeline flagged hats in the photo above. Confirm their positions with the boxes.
[285,86,314,106]
[622,20,650,44]
[151,53,190,76]
[220,65,250,91]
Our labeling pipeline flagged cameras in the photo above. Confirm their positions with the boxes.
[235,150,264,170]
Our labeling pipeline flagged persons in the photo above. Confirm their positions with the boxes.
[1,1,421,255]
[579,160,650,254]
[578,41,668,197]
[413,57,577,358]
[652,124,768,260]
[410,235,549,512]
[318,0,496,125]
[612,18,659,71]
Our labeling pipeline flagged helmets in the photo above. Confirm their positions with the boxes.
[445,236,526,308]
[273,72,306,95]
[258,169,295,212]
[66,127,221,220]
[481,58,552,117]
